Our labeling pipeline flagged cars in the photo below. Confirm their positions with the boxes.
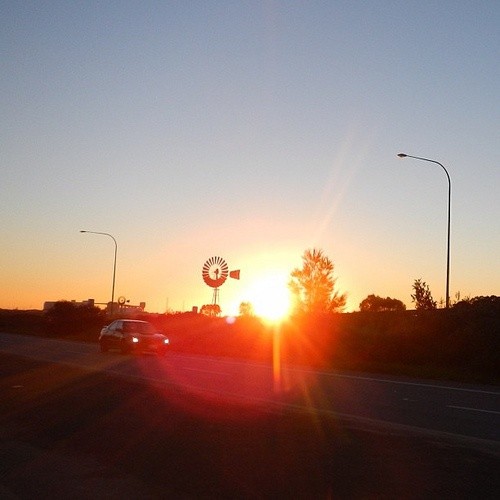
[99,320,170,356]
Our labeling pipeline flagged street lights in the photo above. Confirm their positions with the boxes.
[80,231,117,318]
[397,153,451,307]
